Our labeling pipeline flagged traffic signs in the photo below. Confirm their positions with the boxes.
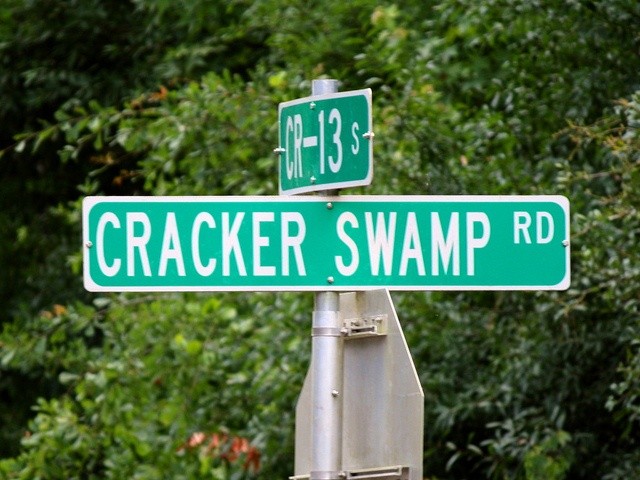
[80,196,573,293]
[276,86,374,196]
